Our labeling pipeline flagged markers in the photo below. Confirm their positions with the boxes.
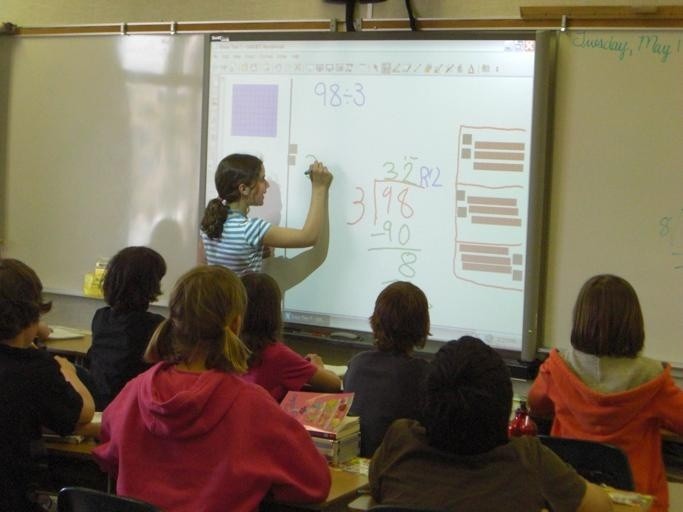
[304,170,311,175]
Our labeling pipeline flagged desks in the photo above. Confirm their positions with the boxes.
[25,320,682,511]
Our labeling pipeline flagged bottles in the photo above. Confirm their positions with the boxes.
[507,399,539,439]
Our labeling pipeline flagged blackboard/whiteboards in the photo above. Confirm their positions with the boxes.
[0,32,206,308]
[540,28,683,367]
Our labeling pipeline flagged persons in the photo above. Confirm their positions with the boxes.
[344,274,682,512]
[199,154,333,278]
[2,246,341,512]
